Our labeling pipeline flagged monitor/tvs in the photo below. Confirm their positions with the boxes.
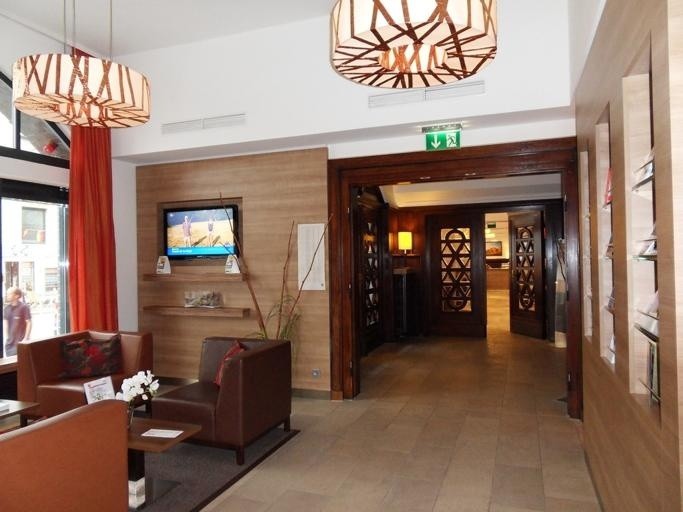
[164,205,238,259]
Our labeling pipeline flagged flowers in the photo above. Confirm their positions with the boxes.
[114,371,159,425]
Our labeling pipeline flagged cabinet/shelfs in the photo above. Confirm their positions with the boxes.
[142,273,249,318]
[631,156,659,401]
[602,200,615,356]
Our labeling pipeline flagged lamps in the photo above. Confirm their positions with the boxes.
[330,0,497,89]
[398,232,412,266]
[12,0,149,129]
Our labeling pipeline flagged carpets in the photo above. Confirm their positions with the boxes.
[0,414,301,512]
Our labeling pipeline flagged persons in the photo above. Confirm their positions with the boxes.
[208,217,214,247]
[4,286,31,357]
[181,216,192,248]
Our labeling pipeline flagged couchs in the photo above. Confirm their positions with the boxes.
[151,336,292,466]
[0,400,129,512]
[17,330,152,426]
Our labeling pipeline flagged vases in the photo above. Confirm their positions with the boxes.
[127,406,135,429]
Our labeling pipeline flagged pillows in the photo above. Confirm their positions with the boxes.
[59,334,123,380]
[213,338,246,386]
